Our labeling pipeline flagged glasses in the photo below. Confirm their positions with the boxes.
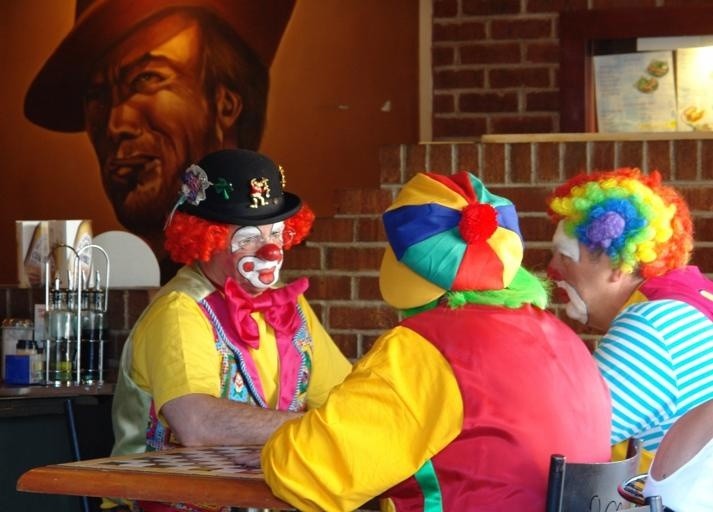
[239,231,295,251]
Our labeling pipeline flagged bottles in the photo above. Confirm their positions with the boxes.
[16,269,108,387]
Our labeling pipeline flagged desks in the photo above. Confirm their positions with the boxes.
[15,446,297,512]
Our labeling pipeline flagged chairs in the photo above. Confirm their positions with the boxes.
[546,437,640,511]
[62,398,115,512]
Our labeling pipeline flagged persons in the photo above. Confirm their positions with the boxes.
[546,163,713,512]
[98,149,354,512]
[20,0,300,286]
[258,171,612,511]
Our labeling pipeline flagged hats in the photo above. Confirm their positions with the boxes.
[172,149,301,226]
[6,0,288,132]
[380,171,524,309]
